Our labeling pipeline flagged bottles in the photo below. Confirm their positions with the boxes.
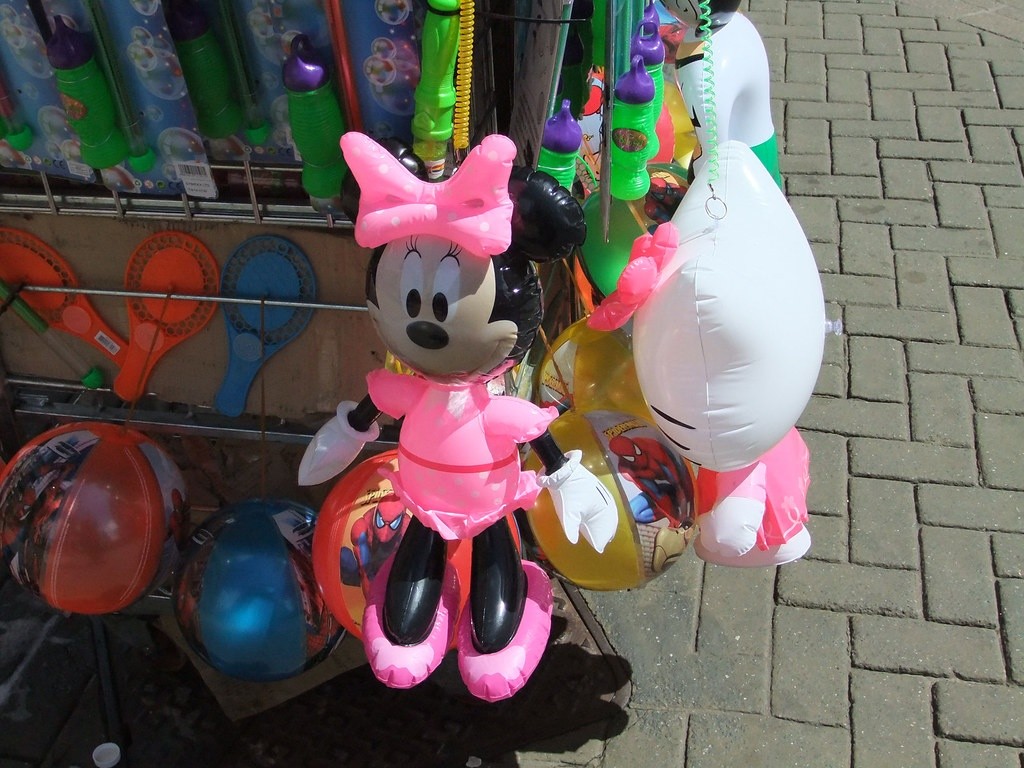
[280,33,348,199]
[163,0,241,140]
[642,0,661,38]
[535,99,583,195]
[611,55,652,201]
[630,21,665,160]
[46,15,131,170]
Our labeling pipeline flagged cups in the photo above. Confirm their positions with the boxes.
[91,742,121,768]
